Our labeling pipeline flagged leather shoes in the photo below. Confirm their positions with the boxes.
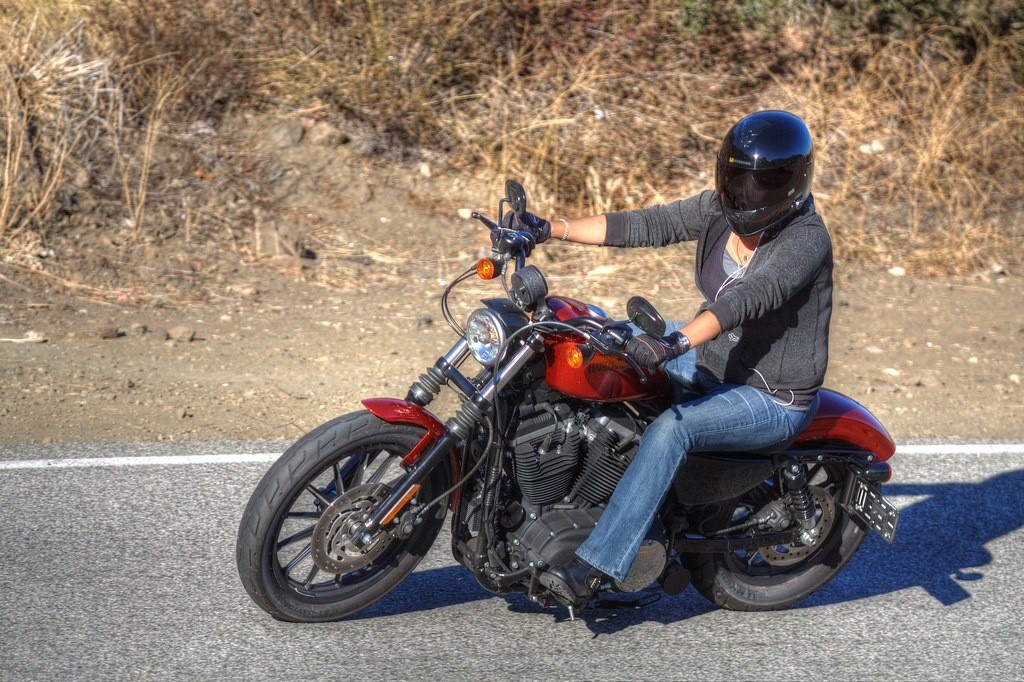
[539,553,608,610]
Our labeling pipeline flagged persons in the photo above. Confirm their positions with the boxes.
[501,110,834,615]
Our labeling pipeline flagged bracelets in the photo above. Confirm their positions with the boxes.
[560,218,570,241]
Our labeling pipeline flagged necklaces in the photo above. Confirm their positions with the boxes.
[741,241,755,261]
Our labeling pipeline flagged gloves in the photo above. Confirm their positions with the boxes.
[623,332,683,375]
[501,208,551,244]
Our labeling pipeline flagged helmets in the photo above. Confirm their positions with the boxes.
[715,110,815,237]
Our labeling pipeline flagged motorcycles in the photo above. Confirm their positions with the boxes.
[233,177,900,625]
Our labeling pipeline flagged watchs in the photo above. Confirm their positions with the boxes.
[671,331,691,354]
